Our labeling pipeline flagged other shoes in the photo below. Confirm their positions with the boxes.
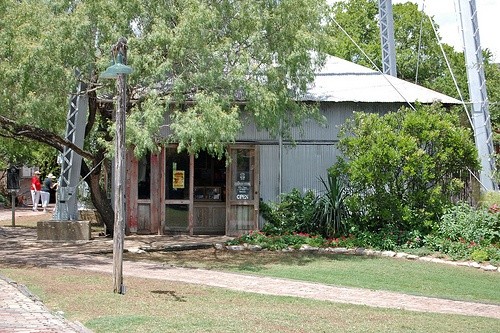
[33,209,38,212]
[43,210,47,212]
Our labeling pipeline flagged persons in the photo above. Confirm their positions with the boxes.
[40,173,58,212]
[30,171,41,211]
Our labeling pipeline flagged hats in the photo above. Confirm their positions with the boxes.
[35,171,41,174]
[46,173,56,178]
